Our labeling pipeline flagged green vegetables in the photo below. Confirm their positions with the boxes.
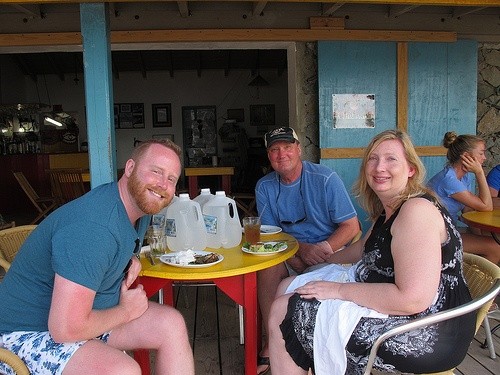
[240,241,285,253]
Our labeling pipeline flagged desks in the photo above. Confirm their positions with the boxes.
[186,166,235,197]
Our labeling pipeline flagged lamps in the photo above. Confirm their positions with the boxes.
[248,61,270,87]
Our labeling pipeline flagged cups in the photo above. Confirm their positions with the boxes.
[243,217,261,243]
[146,225,168,265]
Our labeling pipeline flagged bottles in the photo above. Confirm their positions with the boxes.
[191,188,215,213]
[153,194,178,247]
[202,190,242,249]
[165,194,206,252]
[0,133,41,154]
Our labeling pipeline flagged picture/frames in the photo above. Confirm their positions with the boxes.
[249,104,275,125]
[113,103,145,130]
[227,109,245,123]
[152,103,172,128]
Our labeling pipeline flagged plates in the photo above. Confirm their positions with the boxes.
[242,224,282,235]
[160,251,223,268]
[242,241,287,255]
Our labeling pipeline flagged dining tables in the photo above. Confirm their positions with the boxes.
[460,195,500,234]
[131,231,298,375]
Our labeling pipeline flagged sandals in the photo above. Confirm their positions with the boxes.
[257,355,270,375]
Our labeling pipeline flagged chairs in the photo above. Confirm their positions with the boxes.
[0,225,40,273]
[45,168,85,205]
[233,193,259,217]
[365,252,500,375]
[12,167,65,225]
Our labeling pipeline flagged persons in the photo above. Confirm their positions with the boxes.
[0,140,195,375]
[422,131,500,265]
[268,130,476,375]
[255,126,360,375]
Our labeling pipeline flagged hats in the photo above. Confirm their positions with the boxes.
[264,125,301,151]
